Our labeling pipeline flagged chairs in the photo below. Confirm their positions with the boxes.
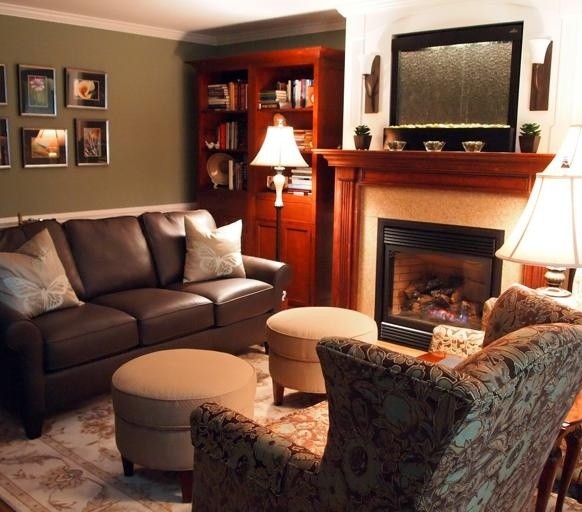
[188,283,581,511]
[427,295,500,358]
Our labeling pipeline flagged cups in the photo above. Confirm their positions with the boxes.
[423,141,446,152]
[461,141,486,152]
[386,142,406,152]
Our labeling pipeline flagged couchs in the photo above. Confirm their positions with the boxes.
[2,208,291,439]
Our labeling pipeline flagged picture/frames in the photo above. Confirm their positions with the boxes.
[73,117,111,166]
[18,63,57,118]
[1,63,9,106]
[21,127,68,168]
[65,68,108,111]
[0,117,12,169]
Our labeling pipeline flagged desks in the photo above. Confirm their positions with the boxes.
[416,350,582,511]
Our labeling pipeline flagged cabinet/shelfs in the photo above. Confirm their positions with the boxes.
[180,46,345,307]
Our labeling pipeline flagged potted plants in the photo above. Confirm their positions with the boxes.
[518,122,541,152]
[353,125,372,151]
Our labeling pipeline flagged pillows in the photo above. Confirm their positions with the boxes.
[181,213,247,284]
[1,228,86,322]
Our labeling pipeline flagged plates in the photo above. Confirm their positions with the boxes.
[207,153,235,188]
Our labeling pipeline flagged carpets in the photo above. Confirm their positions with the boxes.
[2,344,328,511]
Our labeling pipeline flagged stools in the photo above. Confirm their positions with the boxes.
[112,349,257,506]
[265,306,380,407]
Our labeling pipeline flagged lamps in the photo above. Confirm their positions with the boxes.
[248,118,311,263]
[493,158,582,306]
[31,129,67,148]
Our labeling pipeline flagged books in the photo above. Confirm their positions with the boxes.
[206,77,314,197]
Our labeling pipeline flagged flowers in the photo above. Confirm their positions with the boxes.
[72,78,100,100]
[28,76,48,106]
